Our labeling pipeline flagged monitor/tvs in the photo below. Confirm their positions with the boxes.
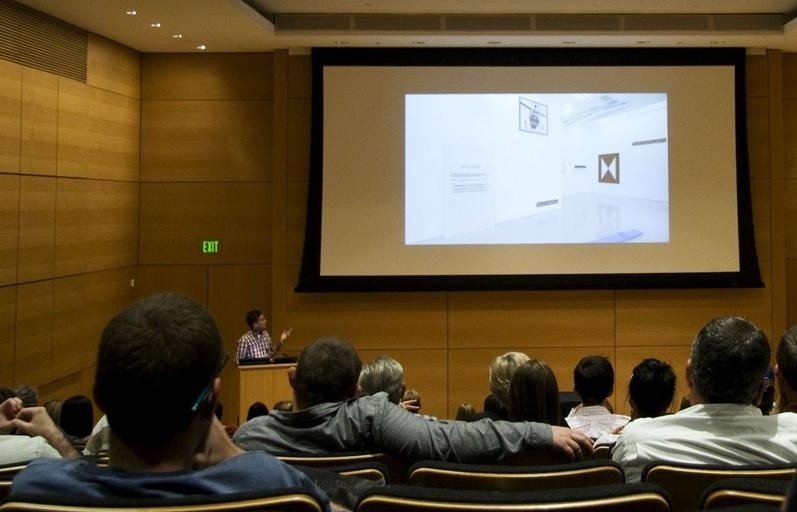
[273,356,297,363]
[239,357,270,365]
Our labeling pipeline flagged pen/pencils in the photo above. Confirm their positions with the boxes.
[191,352,230,413]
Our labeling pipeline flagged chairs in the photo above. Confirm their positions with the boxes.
[0,465,28,505]
[0,494,322,511]
[276,445,796,511]
